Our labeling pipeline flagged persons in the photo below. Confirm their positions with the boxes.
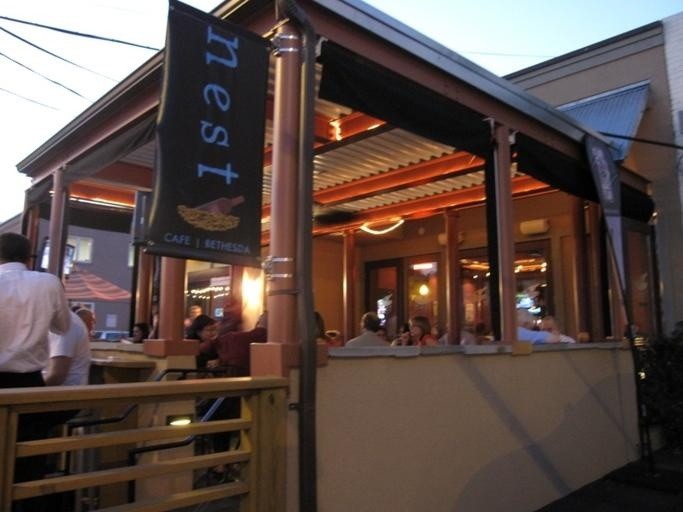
[40,268,93,427]
[130,303,267,482]
[75,308,96,341]
[315,308,577,347]
[0,230,71,512]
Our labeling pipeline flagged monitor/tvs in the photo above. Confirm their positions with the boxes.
[34,236,75,278]
[131,190,154,246]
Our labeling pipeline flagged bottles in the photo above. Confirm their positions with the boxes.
[193,195,245,215]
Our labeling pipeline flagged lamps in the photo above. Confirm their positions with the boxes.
[520,219,550,235]
[167,414,195,429]
[418,276,429,297]
[358,216,406,235]
[437,232,465,245]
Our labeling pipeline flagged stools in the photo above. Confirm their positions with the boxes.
[42,409,120,475]
